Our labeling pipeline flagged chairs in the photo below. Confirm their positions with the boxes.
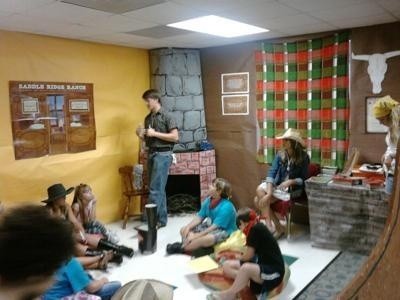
[268,161,324,243]
[117,163,151,230]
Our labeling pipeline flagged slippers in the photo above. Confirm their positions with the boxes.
[206,291,240,300]
[257,287,268,300]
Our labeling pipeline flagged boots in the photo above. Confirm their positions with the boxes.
[84,238,135,265]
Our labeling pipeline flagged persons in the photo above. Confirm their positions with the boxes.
[74,250,112,271]
[135,88,180,230]
[166,177,239,256]
[372,95,400,211]
[67,182,120,244]
[255,127,311,243]
[40,183,134,264]
[206,206,286,300]
[41,215,121,300]
[0,201,77,300]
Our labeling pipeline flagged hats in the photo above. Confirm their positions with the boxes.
[273,127,306,150]
[39,182,75,204]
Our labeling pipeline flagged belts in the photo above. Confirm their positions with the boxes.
[148,146,174,154]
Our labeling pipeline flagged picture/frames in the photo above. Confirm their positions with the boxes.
[363,93,393,135]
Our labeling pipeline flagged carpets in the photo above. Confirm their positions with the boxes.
[83,211,344,300]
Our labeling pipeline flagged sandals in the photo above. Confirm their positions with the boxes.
[95,250,114,270]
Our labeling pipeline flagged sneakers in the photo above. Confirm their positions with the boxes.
[166,241,185,255]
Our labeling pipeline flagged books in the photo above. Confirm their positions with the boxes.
[306,163,385,186]
